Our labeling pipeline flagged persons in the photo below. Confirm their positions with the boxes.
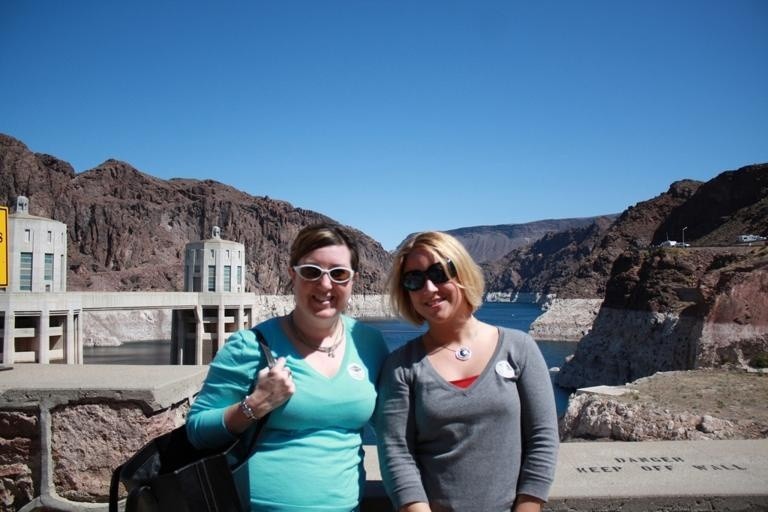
[181,218,392,512]
[370,228,560,511]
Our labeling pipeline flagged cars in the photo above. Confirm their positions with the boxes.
[678,243,690,248]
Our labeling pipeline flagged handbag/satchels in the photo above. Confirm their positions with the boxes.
[110,425,248,512]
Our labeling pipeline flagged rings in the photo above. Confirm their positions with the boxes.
[283,368,292,378]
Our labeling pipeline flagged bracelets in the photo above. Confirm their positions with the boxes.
[240,400,264,426]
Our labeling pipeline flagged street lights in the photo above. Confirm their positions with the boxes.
[683,226,687,248]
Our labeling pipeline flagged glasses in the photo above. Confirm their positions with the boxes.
[402,260,456,291]
[293,264,354,284]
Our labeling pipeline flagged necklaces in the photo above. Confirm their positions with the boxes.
[433,326,482,362]
[287,311,347,359]
